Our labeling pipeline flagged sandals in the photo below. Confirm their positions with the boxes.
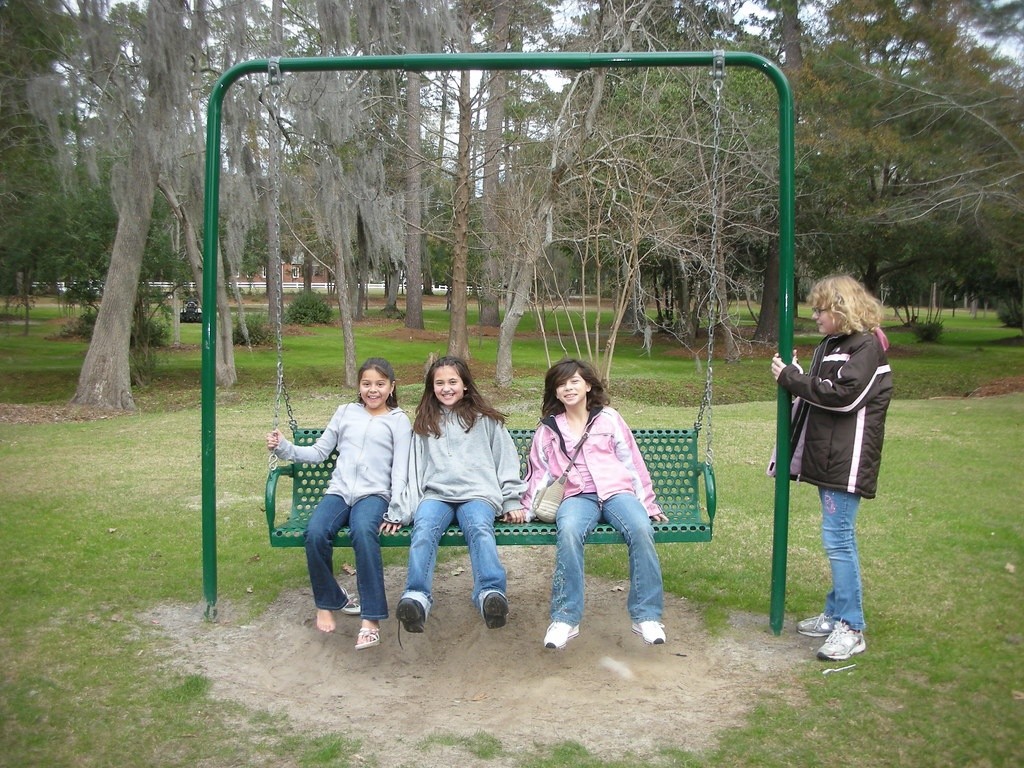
[354,628,380,649]
[341,589,362,614]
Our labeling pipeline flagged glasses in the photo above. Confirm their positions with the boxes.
[812,308,827,317]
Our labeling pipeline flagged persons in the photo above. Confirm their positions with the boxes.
[266,358,412,651]
[505,358,669,649]
[379,356,526,633]
[772,272,892,660]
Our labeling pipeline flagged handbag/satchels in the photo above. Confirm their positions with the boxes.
[534,474,567,523]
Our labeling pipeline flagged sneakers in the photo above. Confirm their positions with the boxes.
[632,620,667,645]
[396,598,425,650]
[543,622,579,650]
[483,592,509,629]
[796,610,837,637]
[817,618,866,661]
